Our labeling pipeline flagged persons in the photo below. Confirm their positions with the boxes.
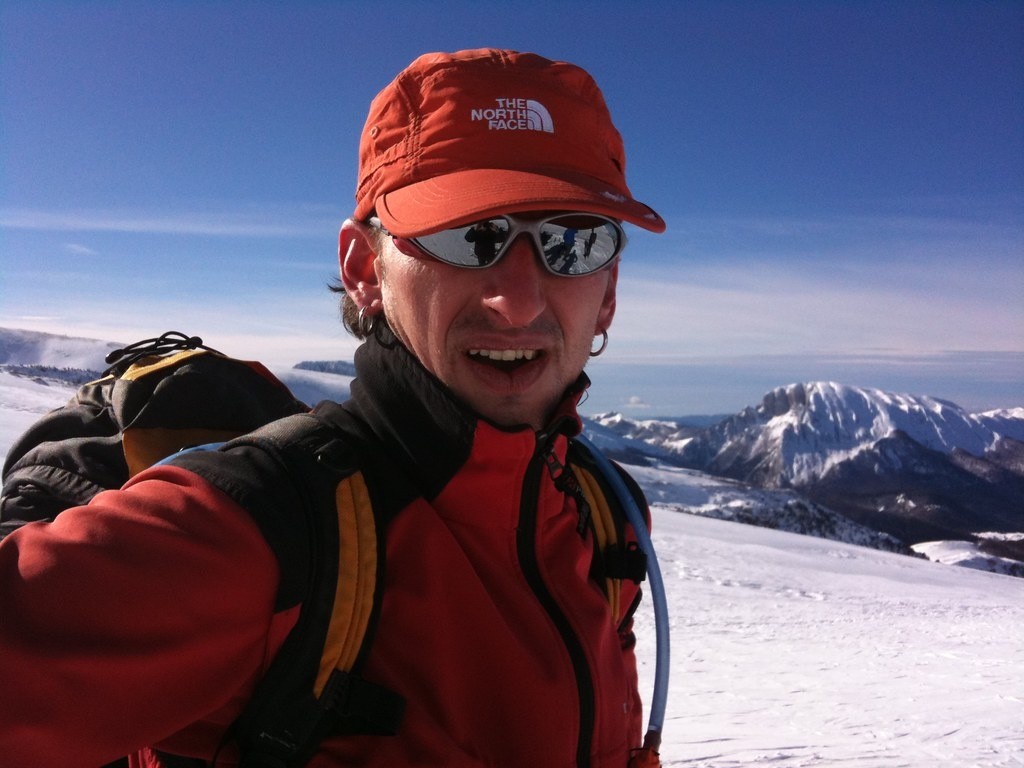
[0,43,674,768]
